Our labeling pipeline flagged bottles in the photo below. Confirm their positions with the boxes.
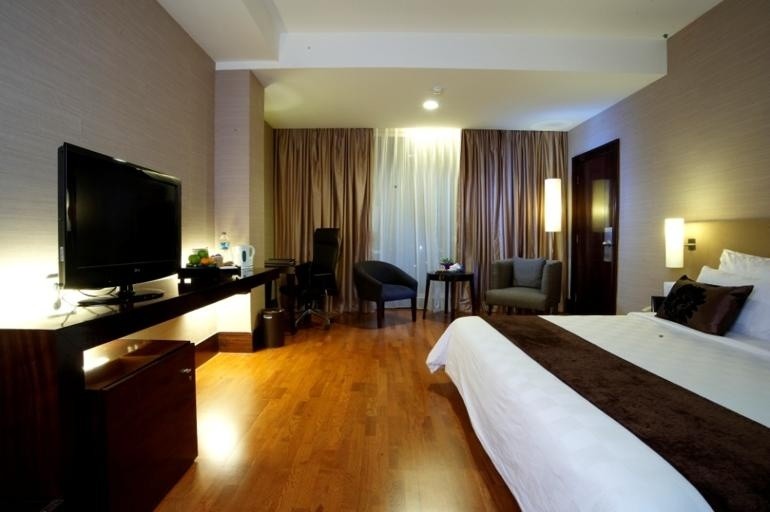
[220,232,230,256]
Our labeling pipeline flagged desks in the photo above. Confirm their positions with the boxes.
[0,267,283,511]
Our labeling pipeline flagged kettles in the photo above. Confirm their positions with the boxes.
[239,245,255,269]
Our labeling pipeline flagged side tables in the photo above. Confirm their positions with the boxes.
[422,272,478,324]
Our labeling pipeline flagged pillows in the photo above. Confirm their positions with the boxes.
[511,255,544,288]
[654,248,770,342]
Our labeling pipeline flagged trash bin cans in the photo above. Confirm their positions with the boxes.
[262,308,285,347]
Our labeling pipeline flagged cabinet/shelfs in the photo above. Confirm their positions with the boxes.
[81,338,197,512]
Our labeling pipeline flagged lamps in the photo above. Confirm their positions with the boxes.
[543,177,564,234]
[663,216,701,268]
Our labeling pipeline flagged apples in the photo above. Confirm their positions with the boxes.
[189,251,216,267]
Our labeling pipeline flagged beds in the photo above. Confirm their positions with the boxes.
[426,310,770,512]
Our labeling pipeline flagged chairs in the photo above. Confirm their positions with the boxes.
[485,259,562,317]
[353,260,418,329]
[290,227,341,334]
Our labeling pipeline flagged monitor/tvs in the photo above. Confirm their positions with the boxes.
[57,141,182,306]
